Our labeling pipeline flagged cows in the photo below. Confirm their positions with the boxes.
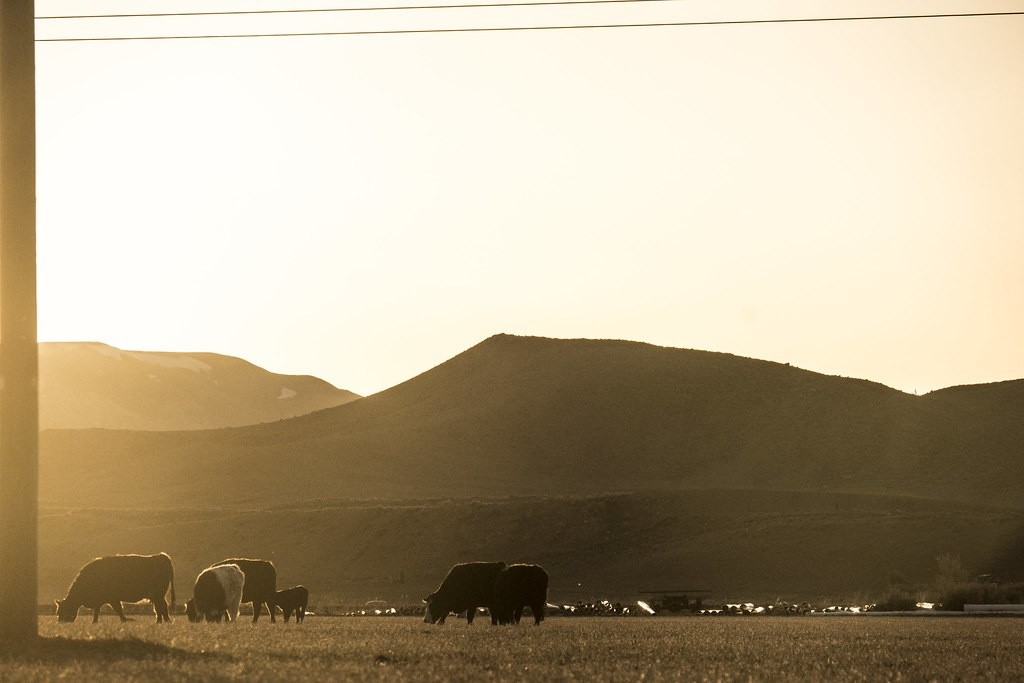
[498,564,549,626]
[55,552,176,623]
[186,558,308,624]
[422,562,509,626]
